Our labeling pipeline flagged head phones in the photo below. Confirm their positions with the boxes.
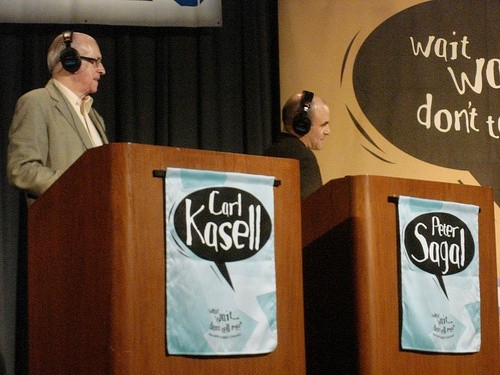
[61,30,81,72]
[292,91,314,135]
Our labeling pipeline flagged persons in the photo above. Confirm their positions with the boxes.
[263,90,331,202]
[6,32,108,207]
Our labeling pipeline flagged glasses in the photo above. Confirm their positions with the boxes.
[80,56,101,67]
